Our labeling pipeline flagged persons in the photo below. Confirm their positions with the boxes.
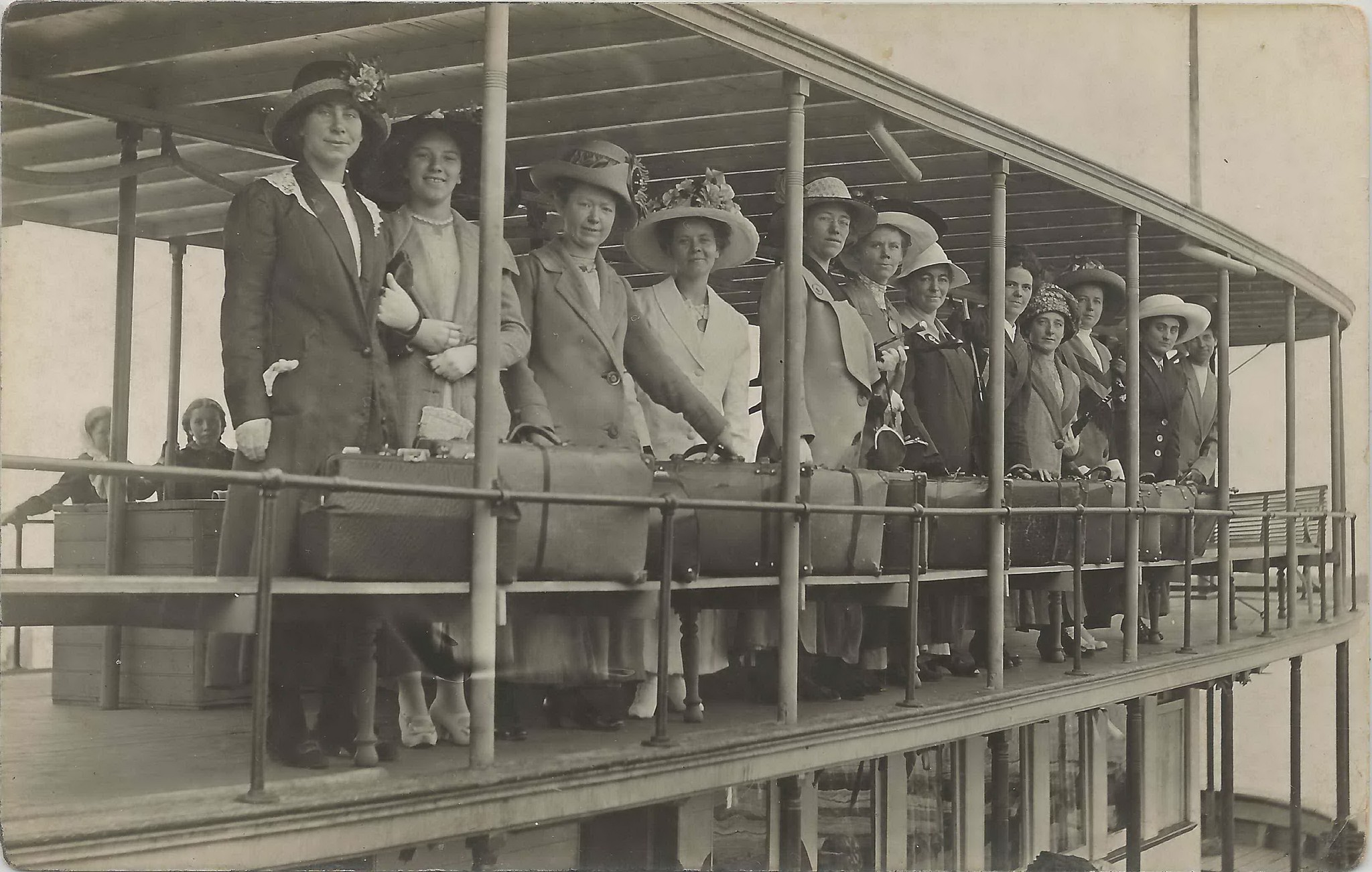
[758,177,880,700]
[623,165,752,720]
[378,107,534,752]
[217,49,422,767]
[0,405,180,529]
[840,196,1219,700]
[499,137,748,743]
[178,397,238,500]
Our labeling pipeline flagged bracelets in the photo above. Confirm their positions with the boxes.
[407,308,423,342]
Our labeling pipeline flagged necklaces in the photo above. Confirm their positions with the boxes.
[407,208,455,226]
[674,280,709,332]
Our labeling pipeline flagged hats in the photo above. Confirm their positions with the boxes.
[1016,281,1081,343]
[895,241,970,290]
[351,109,521,220]
[1112,294,1212,347]
[1054,254,1126,326]
[529,140,639,233]
[261,60,391,162]
[768,171,878,248]
[623,169,761,276]
[836,195,947,273]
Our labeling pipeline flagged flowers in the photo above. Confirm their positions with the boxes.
[339,53,389,103]
[624,155,654,218]
[420,103,484,126]
[658,165,744,214]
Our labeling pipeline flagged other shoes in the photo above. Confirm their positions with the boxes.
[264,602,1163,769]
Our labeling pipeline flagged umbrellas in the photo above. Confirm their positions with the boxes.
[750,339,963,387]
[748,319,928,415]
[1070,380,1124,438]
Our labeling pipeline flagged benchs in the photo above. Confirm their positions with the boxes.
[4,453,1326,800]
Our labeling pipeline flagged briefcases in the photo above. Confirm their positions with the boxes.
[292,448,522,585]
[802,462,889,577]
[1194,482,1223,557]
[878,469,929,575]
[411,423,655,582]
[1150,479,1196,563]
[922,468,1013,568]
[1001,464,1089,567]
[1111,479,1128,562]
[1137,472,1163,563]
[643,445,815,577]
[1078,464,1112,564]
